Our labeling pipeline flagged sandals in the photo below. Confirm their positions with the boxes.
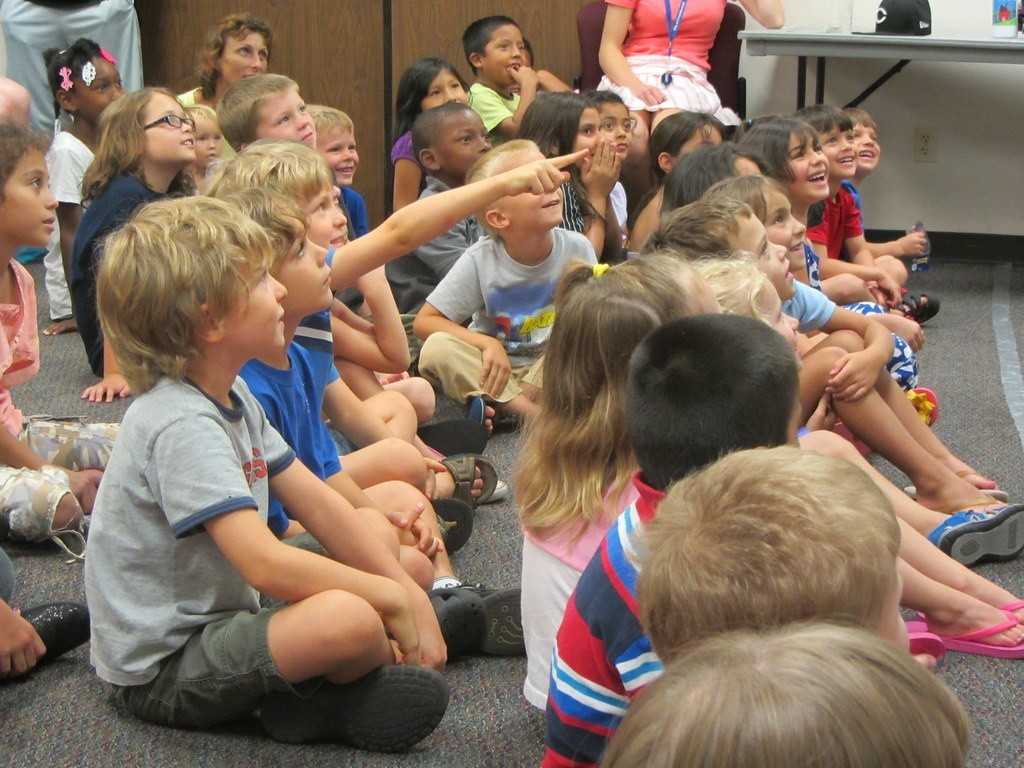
[928,503,1024,567]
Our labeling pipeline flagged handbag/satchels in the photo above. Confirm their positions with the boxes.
[852,0,931,35]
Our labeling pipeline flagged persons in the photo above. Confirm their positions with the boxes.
[84,192,490,754]
[412,138,601,424]
[217,74,317,151]
[392,55,476,212]
[511,103,1024,768]
[0,548,91,687]
[631,442,910,667]
[0,125,121,562]
[463,15,579,135]
[44,11,638,649]
[595,615,970,768]
[0,0,144,143]
[596,0,785,168]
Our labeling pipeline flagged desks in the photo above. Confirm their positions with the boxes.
[734,29,1024,113]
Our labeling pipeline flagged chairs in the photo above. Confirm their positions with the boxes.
[573,2,749,123]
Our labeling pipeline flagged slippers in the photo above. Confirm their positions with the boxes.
[904,472,1008,514]
[905,600,1024,659]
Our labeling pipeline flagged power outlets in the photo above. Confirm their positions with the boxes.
[914,125,938,161]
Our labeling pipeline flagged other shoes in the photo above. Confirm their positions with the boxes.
[20,603,90,665]
[902,294,940,323]
[905,632,946,674]
[430,499,473,556]
[386,588,487,665]
[261,664,450,752]
[467,398,524,440]
[417,418,487,455]
[435,453,497,510]
[452,584,526,656]
[831,388,937,455]
[425,445,508,503]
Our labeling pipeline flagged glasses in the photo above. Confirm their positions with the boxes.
[143,115,196,131]
